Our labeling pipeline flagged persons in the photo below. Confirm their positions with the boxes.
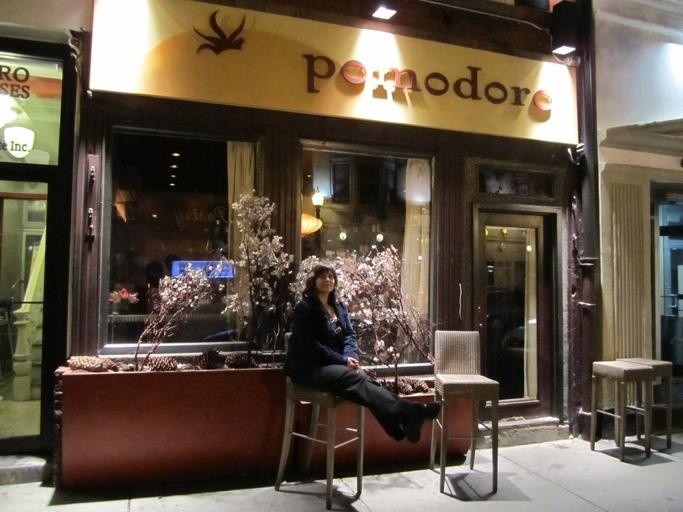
[286,264,441,441]
[127,254,181,341]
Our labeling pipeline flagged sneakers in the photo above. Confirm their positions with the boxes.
[424,400,441,420]
[403,401,423,443]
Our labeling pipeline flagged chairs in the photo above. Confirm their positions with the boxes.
[273,330,370,512]
[423,325,506,497]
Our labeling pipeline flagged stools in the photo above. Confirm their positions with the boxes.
[614,355,672,454]
[587,357,654,467]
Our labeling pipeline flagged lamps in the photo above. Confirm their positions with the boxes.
[548,26,579,58]
[370,0,397,21]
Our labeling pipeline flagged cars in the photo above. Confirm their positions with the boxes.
[200,315,394,341]
